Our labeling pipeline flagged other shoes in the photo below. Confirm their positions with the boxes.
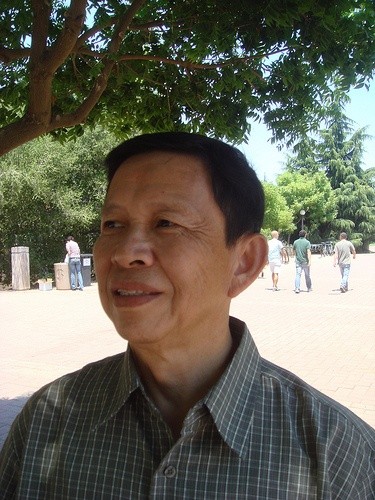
[295,288,300,293]
[308,289,312,292]
[340,287,345,293]
[344,288,348,291]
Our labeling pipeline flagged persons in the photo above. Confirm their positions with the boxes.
[0,132,375,500]
[333,232,356,293]
[66,236,83,291]
[268,231,286,291]
[293,230,311,293]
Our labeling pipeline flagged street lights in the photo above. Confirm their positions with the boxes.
[299,210,305,230]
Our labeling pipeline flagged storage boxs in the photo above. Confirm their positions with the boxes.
[33,278,54,291]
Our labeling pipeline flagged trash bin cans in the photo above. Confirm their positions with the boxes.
[80,254,93,286]
